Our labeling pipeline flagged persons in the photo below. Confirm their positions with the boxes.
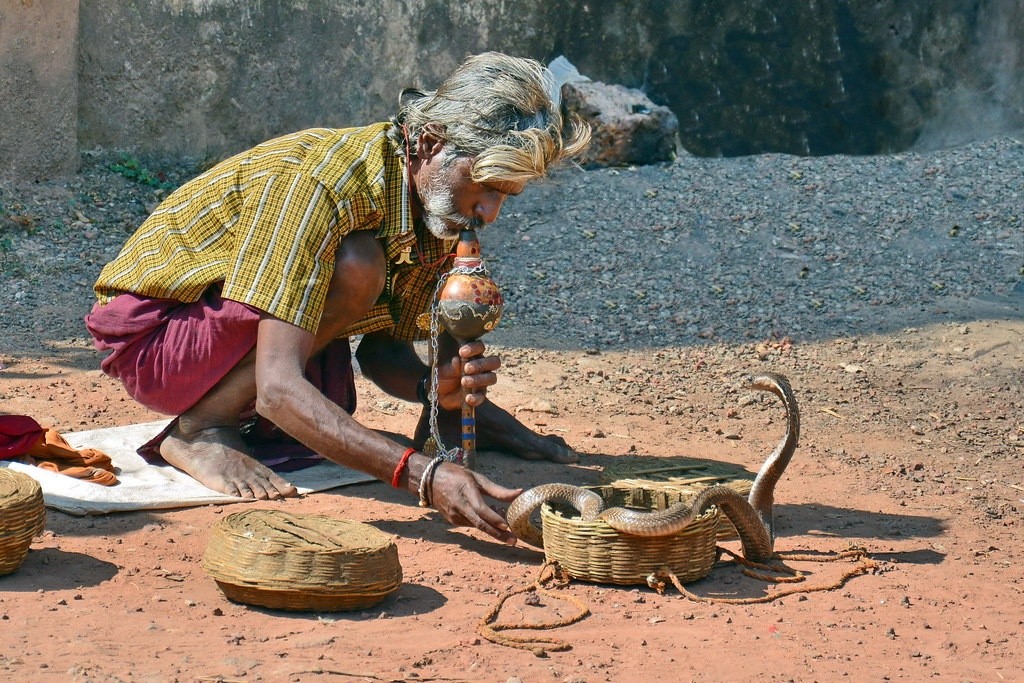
[83,52,578,546]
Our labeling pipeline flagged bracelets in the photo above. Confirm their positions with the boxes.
[419,456,442,508]
[392,447,416,489]
[416,370,432,409]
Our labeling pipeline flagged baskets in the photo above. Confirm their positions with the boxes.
[0,468,45,575]
[541,484,718,583]
[201,508,403,611]
